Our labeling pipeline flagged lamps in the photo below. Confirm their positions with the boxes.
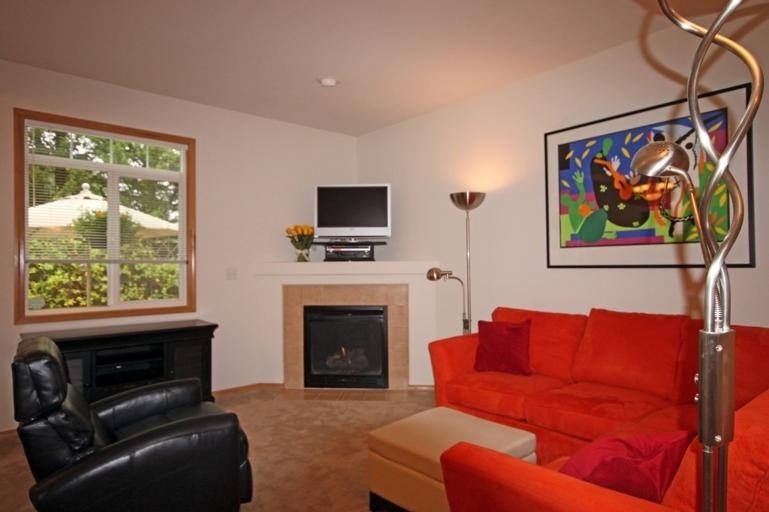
[631,0,764,512]
[427,192,486,335]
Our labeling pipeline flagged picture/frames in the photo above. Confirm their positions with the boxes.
[544,82,755,267]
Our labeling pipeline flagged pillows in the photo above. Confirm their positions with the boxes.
[559,429,690,504]
[474,318,534,375]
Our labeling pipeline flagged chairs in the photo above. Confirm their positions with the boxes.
[12,337,252,512]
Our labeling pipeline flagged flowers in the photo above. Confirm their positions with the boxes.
[285,224,315,242]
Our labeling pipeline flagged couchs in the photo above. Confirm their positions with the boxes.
[428,306,769,512]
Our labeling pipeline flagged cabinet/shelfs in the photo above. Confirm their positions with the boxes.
[19,319,219,404]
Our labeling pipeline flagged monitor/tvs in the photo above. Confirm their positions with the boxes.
[314,185,393,236]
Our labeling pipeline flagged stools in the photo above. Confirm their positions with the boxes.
[367,406,536,512]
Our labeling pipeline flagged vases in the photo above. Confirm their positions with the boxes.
[292,242,313,261]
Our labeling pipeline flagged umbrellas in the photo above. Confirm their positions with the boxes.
[28,183,178,306]
